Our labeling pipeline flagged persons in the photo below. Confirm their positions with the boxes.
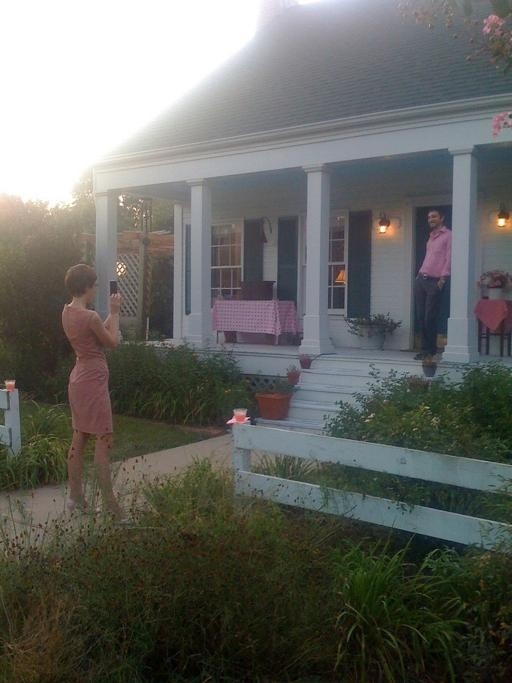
[412,206,453,360]
[61,262,132,519]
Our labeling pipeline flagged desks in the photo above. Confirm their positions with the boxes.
[473,299,512,356]
[209,299,299,345]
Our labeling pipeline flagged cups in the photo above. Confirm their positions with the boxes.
[5,379,15,392]
[233,407,248,421]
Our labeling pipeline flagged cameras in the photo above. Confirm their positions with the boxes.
[110,281,117,296]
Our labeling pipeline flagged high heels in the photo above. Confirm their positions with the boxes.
[66,497,99,516]
[102,503,130,521]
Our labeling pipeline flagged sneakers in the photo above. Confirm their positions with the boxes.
[414,352,436,360]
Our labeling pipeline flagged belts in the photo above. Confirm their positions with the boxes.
[418,274,440,281]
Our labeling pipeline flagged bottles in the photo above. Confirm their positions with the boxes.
[480,279,489,299]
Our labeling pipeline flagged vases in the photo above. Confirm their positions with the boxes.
[488,288,504,301]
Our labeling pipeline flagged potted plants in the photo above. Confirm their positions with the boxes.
[284,359,303,385]
[413,351,438,377]
[251,374,305,420]
[296,350,321,369]
[343,311,404,349]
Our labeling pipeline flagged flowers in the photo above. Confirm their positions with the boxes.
[477,270,512,293]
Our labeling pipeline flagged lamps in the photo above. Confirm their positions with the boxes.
[376,208,401,233]
[487,199,509,228]
[334,270,345,283]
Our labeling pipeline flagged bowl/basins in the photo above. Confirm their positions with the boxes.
[239,280,276,300]
